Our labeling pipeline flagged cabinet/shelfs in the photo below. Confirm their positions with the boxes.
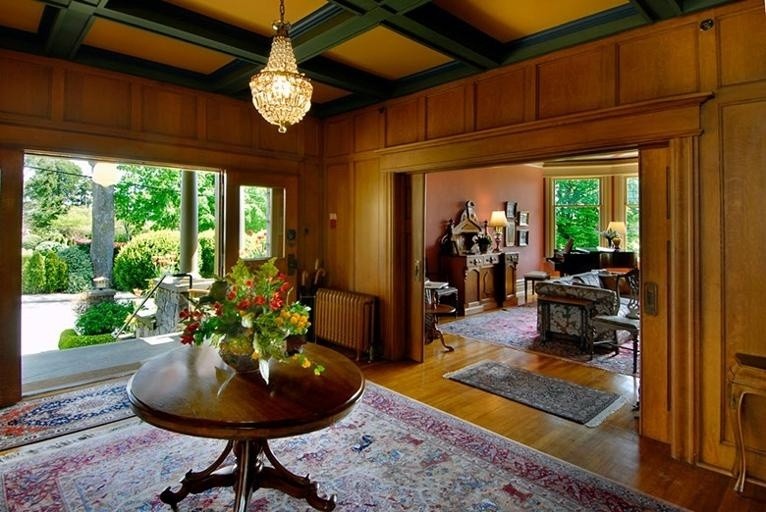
[441,195,522,317]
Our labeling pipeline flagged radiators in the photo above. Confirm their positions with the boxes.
[312,282,379,364]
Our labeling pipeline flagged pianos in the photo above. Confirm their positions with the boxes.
[546,246,634,278]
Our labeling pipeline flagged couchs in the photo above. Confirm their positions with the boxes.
[531,266,640,351]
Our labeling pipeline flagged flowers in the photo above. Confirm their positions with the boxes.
[171,260,333,385]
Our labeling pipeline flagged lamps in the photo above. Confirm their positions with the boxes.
[249,1,315,141]
[486,209,510,251]
[607,219,628,251]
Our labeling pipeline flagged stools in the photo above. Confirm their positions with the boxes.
[524,270,550,304]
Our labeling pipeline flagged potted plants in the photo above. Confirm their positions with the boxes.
[474,235,492,254]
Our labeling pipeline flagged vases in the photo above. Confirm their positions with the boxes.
[214,332,265,375]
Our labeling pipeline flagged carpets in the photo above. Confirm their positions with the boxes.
[440,278,642,381]
[444,356,628,432]
[1,375,699,511]
[0,380,149,453]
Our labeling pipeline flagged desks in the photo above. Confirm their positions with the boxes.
[718,349,764,496]
[425,301,456,356]
[126,336,364,510]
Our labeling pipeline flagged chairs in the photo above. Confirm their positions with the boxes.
[590,267,640,374]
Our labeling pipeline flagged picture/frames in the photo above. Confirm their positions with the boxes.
[503,200,519,218]
[503,221,519,247]
[517,210,530,227]
[517,228,530,247]
